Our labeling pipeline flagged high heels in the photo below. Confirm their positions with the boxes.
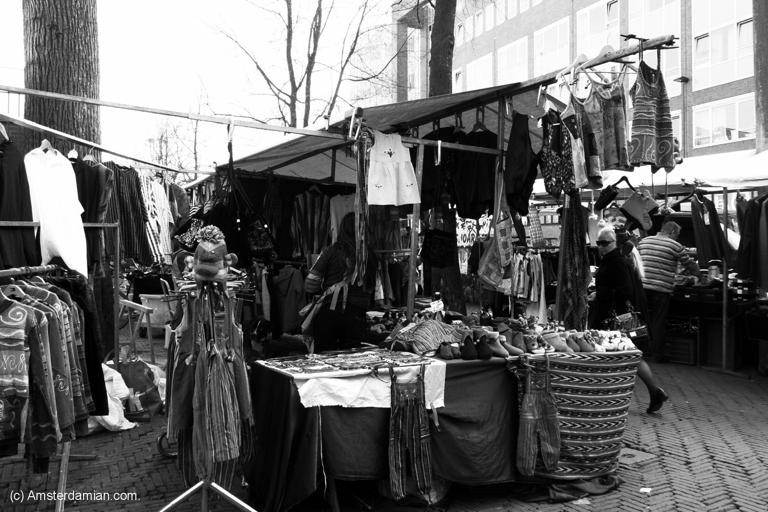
[648,386,669,412]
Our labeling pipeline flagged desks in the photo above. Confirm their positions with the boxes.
[255,345,546,512]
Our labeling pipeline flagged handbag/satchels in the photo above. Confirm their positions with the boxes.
[612,308,650,343]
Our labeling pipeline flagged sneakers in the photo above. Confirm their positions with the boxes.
[439,341,461,360]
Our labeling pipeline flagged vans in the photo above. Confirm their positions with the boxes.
[479,209,562,247]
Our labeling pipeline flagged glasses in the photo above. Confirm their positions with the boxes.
[595,240,613,247]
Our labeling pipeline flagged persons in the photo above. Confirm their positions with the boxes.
[635,221,709,365]
[587,225,669,415]
[302,210,387,356]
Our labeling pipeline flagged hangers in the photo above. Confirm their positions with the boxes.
[556,176,748,215]
[0,121,259,304]
[536,33,680,109]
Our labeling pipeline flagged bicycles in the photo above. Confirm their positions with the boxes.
[118,257,165,329]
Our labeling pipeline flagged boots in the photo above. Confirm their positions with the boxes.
[462,329,607,359]
